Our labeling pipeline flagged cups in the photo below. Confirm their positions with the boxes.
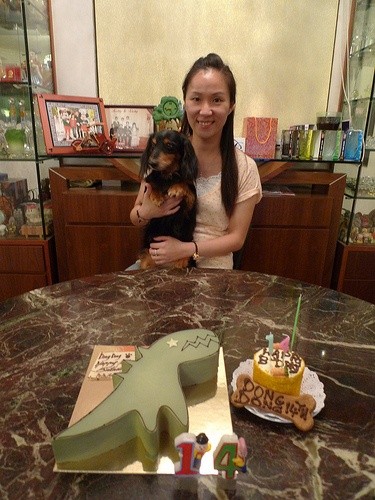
[322,130,343,161]
[344,130,364,162]
[289,124,315,134]
[281,129,298,158]
[299,130,322,160]
[316,111,342,131]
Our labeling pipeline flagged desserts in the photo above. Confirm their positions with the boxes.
[253,343,305,396]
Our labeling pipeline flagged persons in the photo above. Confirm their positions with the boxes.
[57,111,141,148]
[125,54,263,274]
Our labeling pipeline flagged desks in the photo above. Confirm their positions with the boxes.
[0,264,375,500]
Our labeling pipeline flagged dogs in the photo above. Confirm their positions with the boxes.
[138,129,199,272]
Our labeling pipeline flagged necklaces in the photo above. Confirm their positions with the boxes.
[198,151,219,176]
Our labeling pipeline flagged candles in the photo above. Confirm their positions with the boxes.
[172,428,206,477]
[213,433,248,480]
[278,332,290,352]
[265,330,274,352]
[290,292,302,353]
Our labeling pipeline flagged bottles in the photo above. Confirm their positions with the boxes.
[2,96,35,157]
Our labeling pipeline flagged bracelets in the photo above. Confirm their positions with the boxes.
[137,207,149,224]
[192,240,200,261]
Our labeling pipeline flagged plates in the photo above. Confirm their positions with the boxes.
[234,360,322,423]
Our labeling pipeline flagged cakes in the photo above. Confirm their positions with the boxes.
[51,328,221,472]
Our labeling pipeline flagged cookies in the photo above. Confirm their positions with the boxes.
[230,373,316,431]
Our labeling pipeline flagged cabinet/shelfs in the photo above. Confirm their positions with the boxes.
[0,0,375,305]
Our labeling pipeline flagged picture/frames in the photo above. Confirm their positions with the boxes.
[35,92,108,155]
[104,102,157,154]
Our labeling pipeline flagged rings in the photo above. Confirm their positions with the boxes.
[155,249,158,256]
